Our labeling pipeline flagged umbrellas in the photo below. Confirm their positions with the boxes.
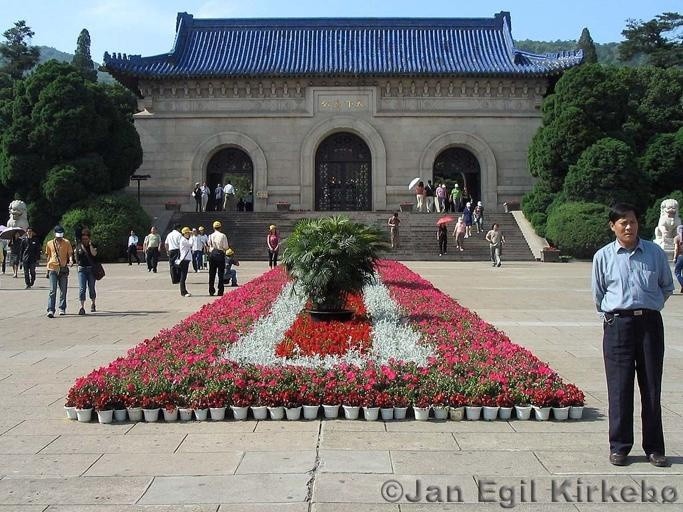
[0,226,26,239]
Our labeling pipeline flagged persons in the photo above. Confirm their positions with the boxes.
[1,239,8,274]
[46,226,72,317]
[192,180,253,212]
[409,178,485,260]
[0,241,6,273]
[74,230,100,315]
[673,225,683,294]
[20,227,40,288]
[128,222,239,297]
[388,212,401,248]
[267,224,279,269]
[485,220,507,267]
[593,202,674,467]
[8,231,23,279]
[0,225,11,232]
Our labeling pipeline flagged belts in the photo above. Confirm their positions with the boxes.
[603,309,661,317]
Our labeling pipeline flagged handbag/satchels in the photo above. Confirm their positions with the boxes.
[173,259,184,269]
[92,263,105,280]
[59,266,70,278]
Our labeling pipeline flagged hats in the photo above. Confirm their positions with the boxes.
[212,221,221,228]
[226,248,234,255]
[269,224,276,232]
[181,226,192,233]
[477,200,482,207]
[54,226,64,238]
[198,227,205,230]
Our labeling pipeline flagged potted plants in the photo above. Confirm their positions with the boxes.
[275,212,397,324]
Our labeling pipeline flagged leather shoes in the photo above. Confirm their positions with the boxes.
[610,451,629,467]
[646,452,669,467]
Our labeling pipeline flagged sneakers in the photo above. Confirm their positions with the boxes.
[47,311,54,318]
[90,304,95,312]
[59,309,65,315]
[79,308,86,315]
